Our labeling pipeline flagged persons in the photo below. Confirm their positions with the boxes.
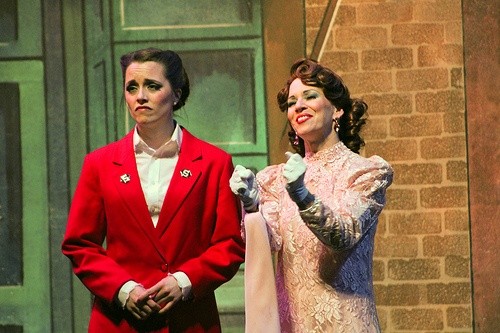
[228,58,394,333]
[61,47,248,333]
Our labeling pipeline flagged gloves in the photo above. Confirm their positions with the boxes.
[228,164,262,212]
[282,151,313,208]
